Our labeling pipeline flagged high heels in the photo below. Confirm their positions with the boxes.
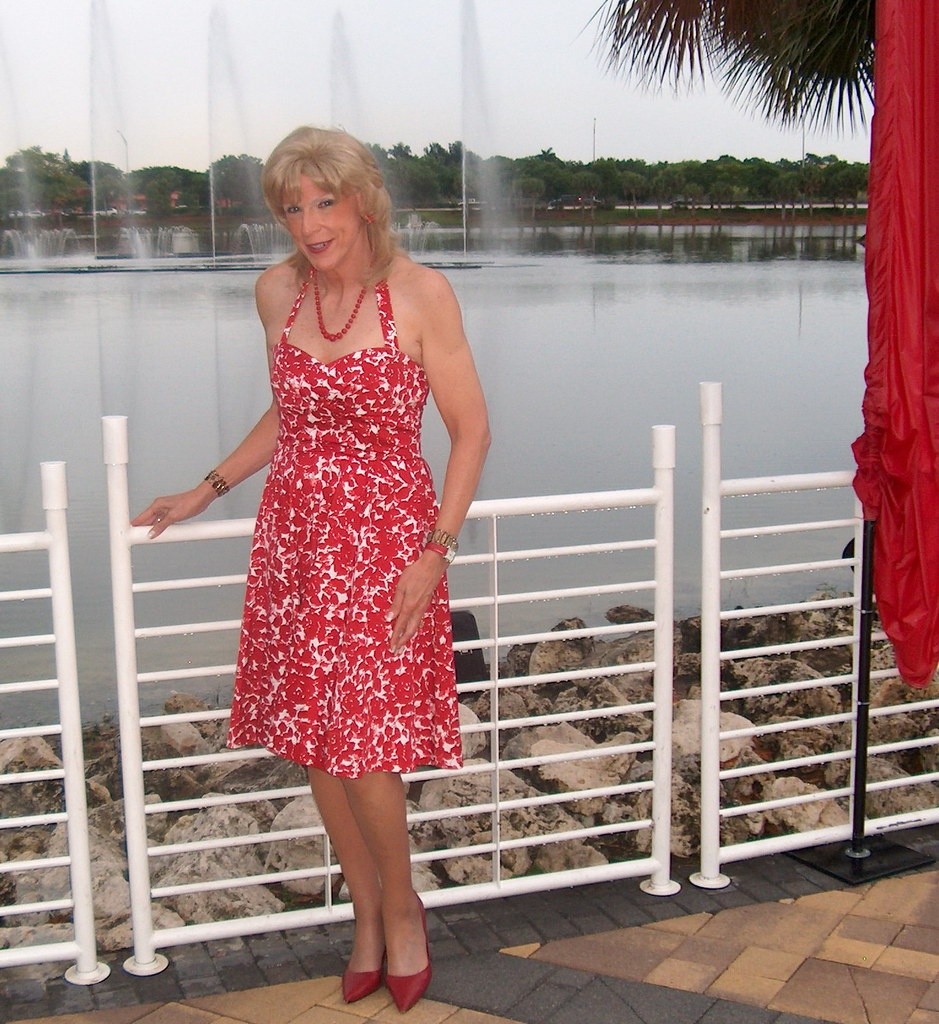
[384,889,431,1013]
[342,944,387,1003]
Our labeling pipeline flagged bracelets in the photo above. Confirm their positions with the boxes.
[427,529,459,551]
[206,471,229,496]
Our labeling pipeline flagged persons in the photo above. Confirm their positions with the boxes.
[131,125,492,1013]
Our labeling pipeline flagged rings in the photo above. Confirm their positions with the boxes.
[157,518,161,523]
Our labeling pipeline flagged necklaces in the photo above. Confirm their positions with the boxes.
[312,270,367,341]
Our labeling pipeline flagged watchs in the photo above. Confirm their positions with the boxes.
[426,543,455,564]
